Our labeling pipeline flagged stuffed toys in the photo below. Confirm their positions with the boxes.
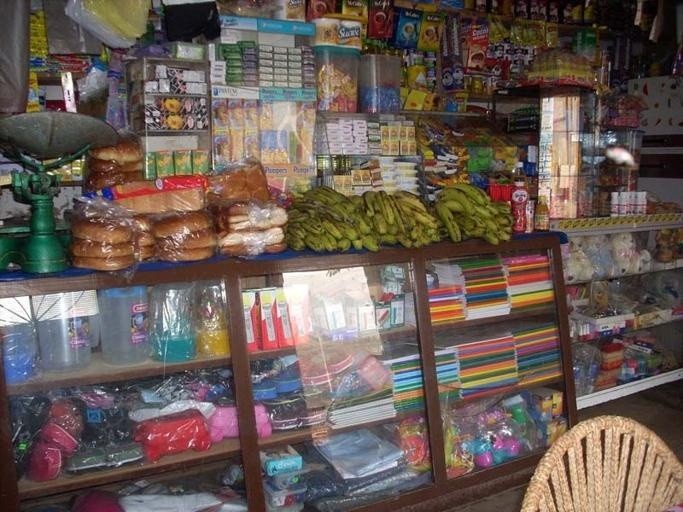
[560,237,594,285]
[609,232,651,276]
[583,235,619,280]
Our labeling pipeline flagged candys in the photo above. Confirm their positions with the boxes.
[359,84,400,114]
[318,65,357,113]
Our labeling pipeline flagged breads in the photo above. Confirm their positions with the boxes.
[86,159,144,177]
[210,163,270,203]
[72,220,133,244]
[217,204,287,229]
[87,177,206,211]
[87,173,144,190]
[88,137,141,164]
[72,241,134,259]
[156,212,213,235]
[130,215,154,231]
[71,256,136,272]
[218,229,285,256]
[135,233,154,247]
[156,230,218,249]
[157,249,216,260]
[134,247,154,260]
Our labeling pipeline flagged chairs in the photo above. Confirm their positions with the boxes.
[520,415,682,512]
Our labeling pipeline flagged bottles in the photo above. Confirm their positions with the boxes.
[626,193,630,215]
[535,197,550,232]
[619,192,626,218]
[511,182,527,231]
[630,192,636,215]
[642,192,647,214]
[610,192,619,219]
[513,162,527,190]
[636,191,644,214]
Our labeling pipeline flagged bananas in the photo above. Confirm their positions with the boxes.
[280,187,381,253]
[427,184,514,246]
[348,189,439,249]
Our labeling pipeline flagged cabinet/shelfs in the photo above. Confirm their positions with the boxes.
[0,235,576,512]
[548,214,683,410]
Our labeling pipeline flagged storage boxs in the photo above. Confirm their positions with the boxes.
[260,88,317,165]
[593,168,639,217]
[538,175,593,218]
[540,133,594,176]
[126,56,210,95]
[134,131,211,180]
[129,93,210,134]
[209,15,259,87]
[594,127,646,167]
[311,45,361,111]
[540,86,596,133]
[211,86,259,165]
[257,18,316,89]
[359,48,403,112]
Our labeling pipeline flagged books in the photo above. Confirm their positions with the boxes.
[372,344,463,412]
[326,387,398,431]
[489,317,564,387]
[431,256,512,320]
[404,326,520,400]
[427,284,466,325]
[499,251,556,315]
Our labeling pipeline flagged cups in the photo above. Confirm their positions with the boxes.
[101,286,149,365]
[2,297,37,384]
[150,284,193,361]
[196,279,229,358]
[33,291,100,372]
